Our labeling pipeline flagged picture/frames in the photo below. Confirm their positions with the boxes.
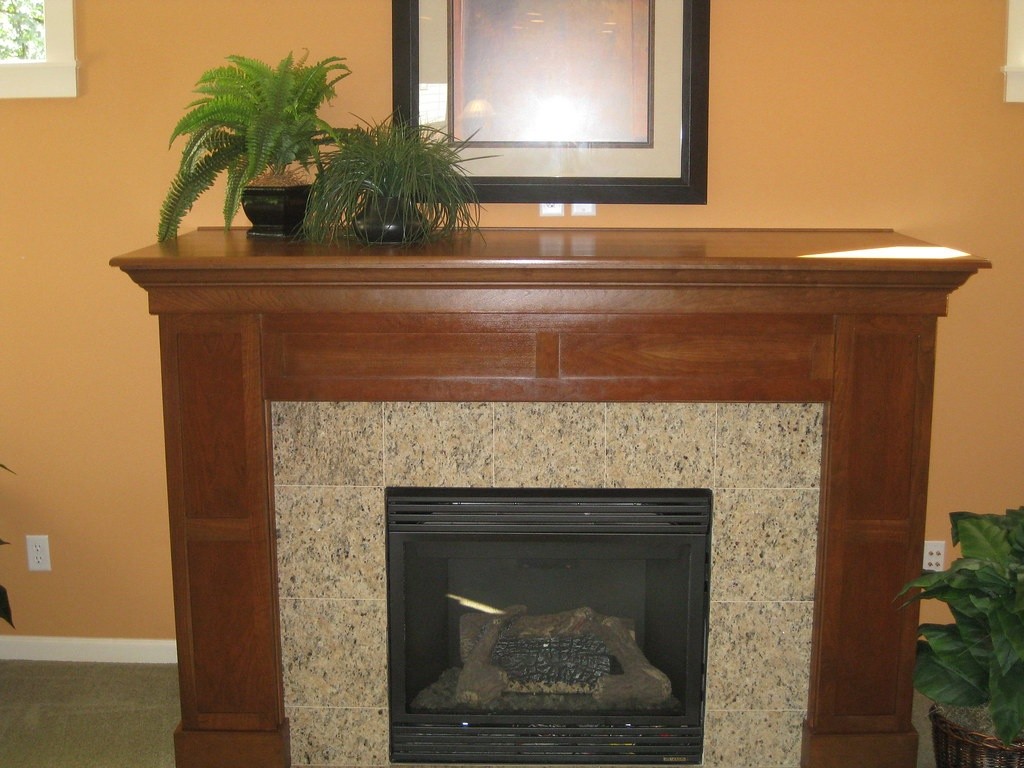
[392,0,712,205]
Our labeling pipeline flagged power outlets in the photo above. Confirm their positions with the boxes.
[26,535,52,571]
[924,540,945,572]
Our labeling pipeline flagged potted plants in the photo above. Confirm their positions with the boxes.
[892,506,1024,768]
[155,48,357,245]
[297,105,504,248]
